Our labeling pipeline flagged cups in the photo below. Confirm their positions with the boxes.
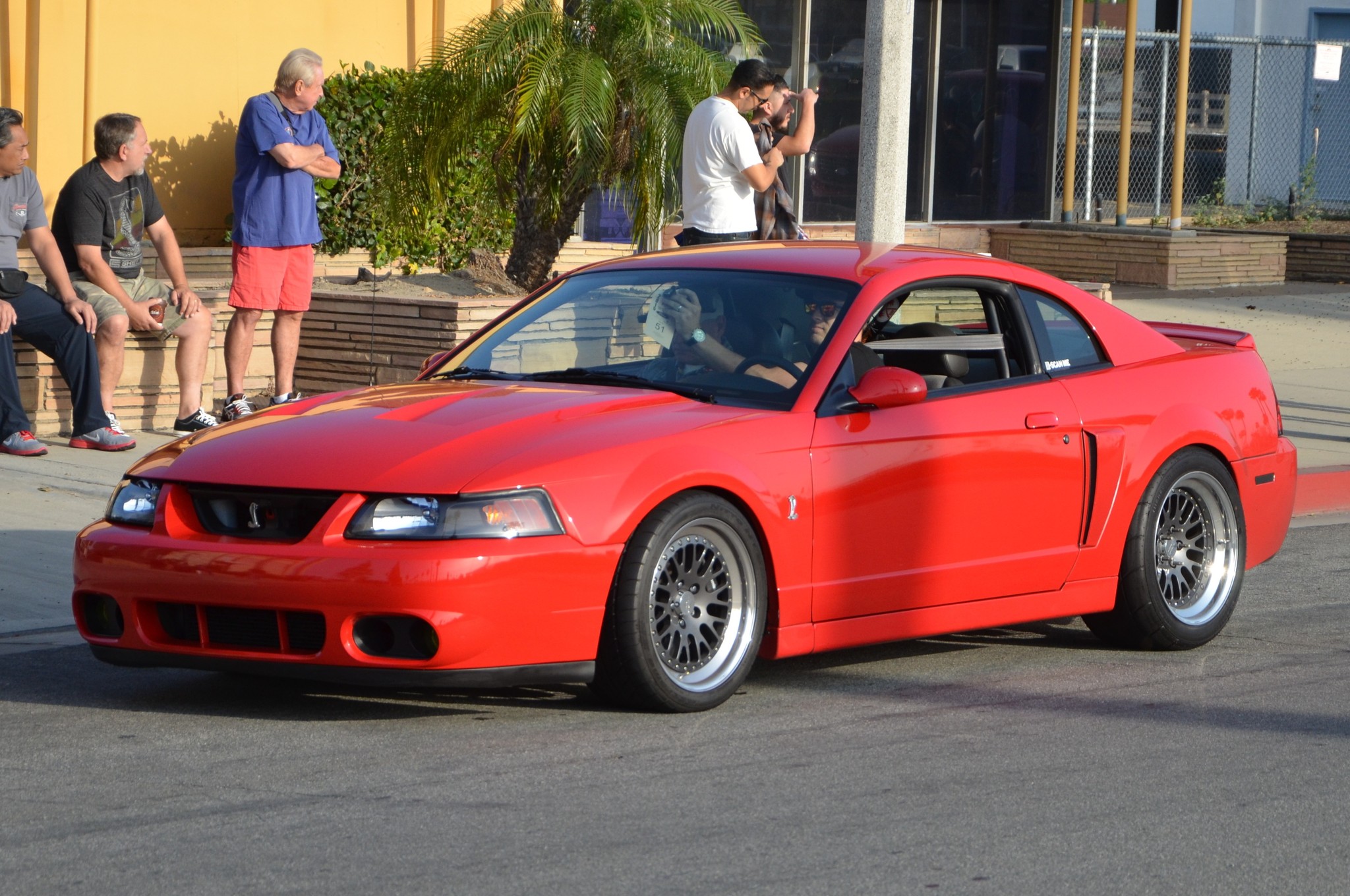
[148,297,168,326]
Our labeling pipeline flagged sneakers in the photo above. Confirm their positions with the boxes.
[221,393,253,423]
[68,426,136,452]
[102,412,130,437]
[172,408,218,437]
[268,392,303,405]
[0,431,48,455]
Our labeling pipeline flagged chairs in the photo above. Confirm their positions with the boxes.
[722,316,787,358]
[884,323,970,395]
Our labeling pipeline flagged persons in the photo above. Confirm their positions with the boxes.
[0,105,136,456]
[681,57,785,248]
[220,49,340,423]
[973,107,1037,191]
[638,286,881,410]
[747,72,818,197]
[44,113,219,437]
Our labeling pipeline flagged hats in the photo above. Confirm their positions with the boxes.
[795,280,862,309]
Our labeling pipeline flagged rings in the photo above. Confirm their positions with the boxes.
[676,306,682,312]
[194,300,198,302]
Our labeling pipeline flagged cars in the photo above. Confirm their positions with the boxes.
[723,39,830,107]
[73,239,1297,714]
[995,44,1049,72]
[807,40,865,111]
[807,70,1045,202]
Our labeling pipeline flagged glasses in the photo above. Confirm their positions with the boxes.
[804,301,843,318]
[750,90,767,106]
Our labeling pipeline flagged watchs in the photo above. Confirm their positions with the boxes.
[685,327,706,348]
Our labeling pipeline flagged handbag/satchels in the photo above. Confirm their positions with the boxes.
[0,268,28,297]
